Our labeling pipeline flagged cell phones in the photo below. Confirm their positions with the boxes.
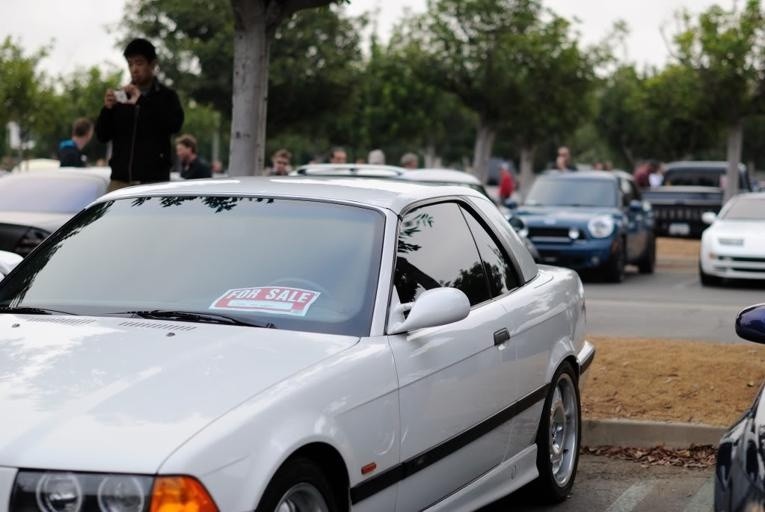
[114,89,129,105]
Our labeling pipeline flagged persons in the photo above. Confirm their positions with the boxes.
[57,117,93,168]
[593,161,614,171]
[401,153,418,168]
[329,147,347,164]
[210,159,224,175]
[634,159,655,185]
[496,161,517,206]
[301,144,326,165]
[172,135,213,178]
[95,37,185,198]
[368,148,388,165]
[264,149,292,178]
[553,146,575,173]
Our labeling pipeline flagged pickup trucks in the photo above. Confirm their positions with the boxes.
[652,161,754,238]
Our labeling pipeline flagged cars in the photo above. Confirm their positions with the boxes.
[0,165,233,283]
[700,193,765,289]
[289,162,540,261]
[713,302,765,512]
[508,168,658,281]
[0,174,594,511]
